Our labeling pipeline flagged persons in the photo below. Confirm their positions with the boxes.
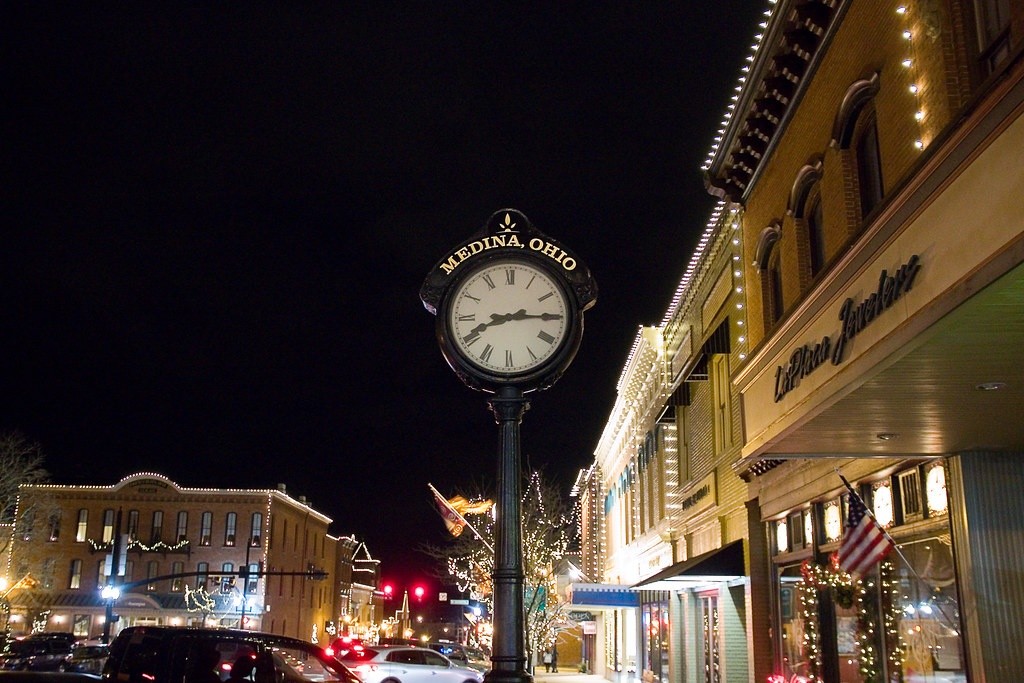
[521,657,535,683]
[543,647,559,673]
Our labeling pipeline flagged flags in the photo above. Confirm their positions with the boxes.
[837,489,896,584]
[435,492,492,538]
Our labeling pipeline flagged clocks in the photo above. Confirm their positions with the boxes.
[449,260,568,373]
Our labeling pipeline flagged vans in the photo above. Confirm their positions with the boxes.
[102,625,365,683]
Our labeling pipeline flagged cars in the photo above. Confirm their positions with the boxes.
[323,644,485,683]
[324,637,493,670]
[0,629,112,683]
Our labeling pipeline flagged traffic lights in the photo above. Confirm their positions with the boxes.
[414,587,424,596]
[384,585,393,597]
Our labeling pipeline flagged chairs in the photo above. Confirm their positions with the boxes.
[185,649,224,683]
[224,656,255,683]
